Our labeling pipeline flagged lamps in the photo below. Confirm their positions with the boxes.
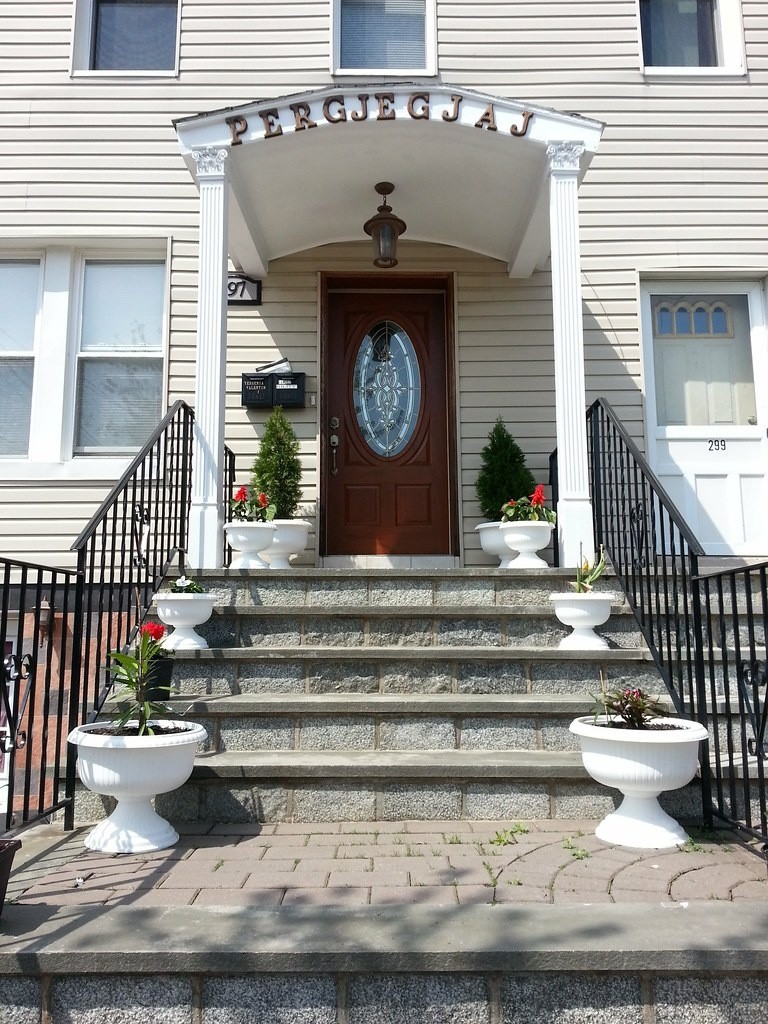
[363,181,406,267]
[30,595,58,649]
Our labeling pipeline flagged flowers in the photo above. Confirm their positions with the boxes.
[168,575,204,593]
[99,621,180,736]
[588,668,670,729]
[498,483,557,524]
[568,541,607,593]
[229,485,277,522]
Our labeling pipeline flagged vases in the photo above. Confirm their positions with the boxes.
[151,593,218,650]
[568,714,709,849]
[0,838,22,916]
[66,720,208,854]
[499,521,554,568]
[549,594,615,650]
[223,521,277,569]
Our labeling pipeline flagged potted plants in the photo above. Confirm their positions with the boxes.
[473,413,538,568]
[246,404,312,569]
